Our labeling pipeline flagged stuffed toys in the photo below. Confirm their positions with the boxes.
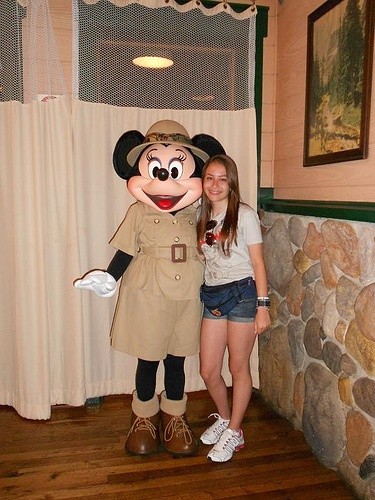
[74,121,227,460]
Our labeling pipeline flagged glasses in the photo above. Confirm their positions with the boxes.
[205,220,217,247]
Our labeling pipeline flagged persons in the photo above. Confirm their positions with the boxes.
[196,155,271,463]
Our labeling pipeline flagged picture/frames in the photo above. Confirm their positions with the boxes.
[302,0,375,167]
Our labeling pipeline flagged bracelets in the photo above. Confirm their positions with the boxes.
[256,297,270,307]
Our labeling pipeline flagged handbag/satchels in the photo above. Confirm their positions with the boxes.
[200,277,254,317]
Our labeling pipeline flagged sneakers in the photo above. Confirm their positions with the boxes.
[206,429,246,463]
[199,413,230,445]
